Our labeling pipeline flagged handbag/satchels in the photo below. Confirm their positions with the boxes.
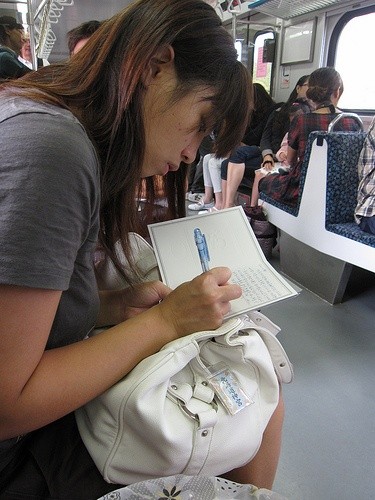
[75,231,294,485]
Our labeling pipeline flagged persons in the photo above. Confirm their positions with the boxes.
[243,65,360,222]
[185,75,315,215]
[353,112,375,236]
[17,30,50,72]
[0,0,287,500]
[0,15,36,83]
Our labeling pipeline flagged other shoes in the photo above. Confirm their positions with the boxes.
[185,192,204,202]
[278,168,289,176]
[188,201,214,210]
[198,206,218,214]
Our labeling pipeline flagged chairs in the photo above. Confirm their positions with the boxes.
[258,131,375,306]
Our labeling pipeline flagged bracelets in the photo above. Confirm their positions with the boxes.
[263,161,272,168]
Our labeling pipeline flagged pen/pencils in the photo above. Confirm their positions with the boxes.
[194,228,211,273]
